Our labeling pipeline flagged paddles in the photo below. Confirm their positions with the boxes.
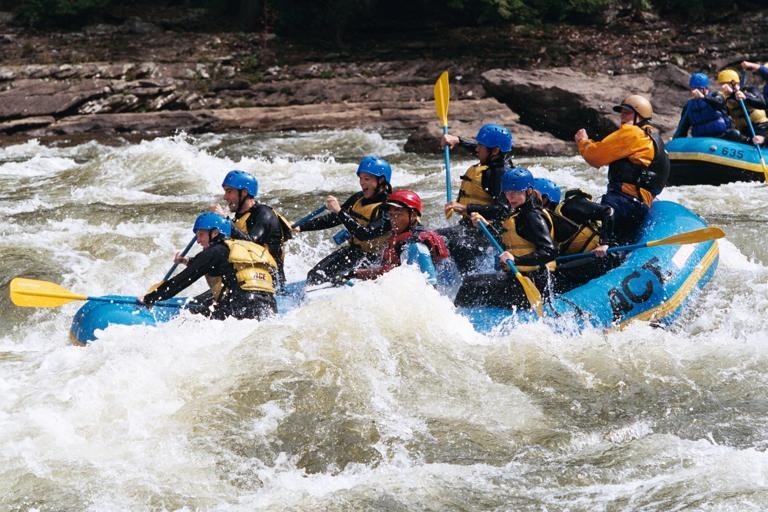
[8,277,209,309]
[475,216,541,316]
[557,227,725,263]
[434,70,450,203]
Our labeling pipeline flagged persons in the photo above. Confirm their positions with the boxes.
[671,71,749,146]
[208,170,286,294]
[453,167,555,310]
[332,190,447,290]
[137,212,277,319]
[432,122,515,269]
[286,155,391,286]
[535,178,631,285]
[574,94,670,250]
[716,69,768,149]
[739,58,767,110]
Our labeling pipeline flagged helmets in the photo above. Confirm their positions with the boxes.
[718,69,741,84]
[501,166,535,193]
[611,94,654,122]
[532,177,561,203]
[222,169,259,197]
[356,155,392,183]
[192,211,232,239]
[389,189,422,214]
[477,124,511,153]
[689,71,709,90]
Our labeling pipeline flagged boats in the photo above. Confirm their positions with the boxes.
[59,194,731,359]
[653,135,768,188]
